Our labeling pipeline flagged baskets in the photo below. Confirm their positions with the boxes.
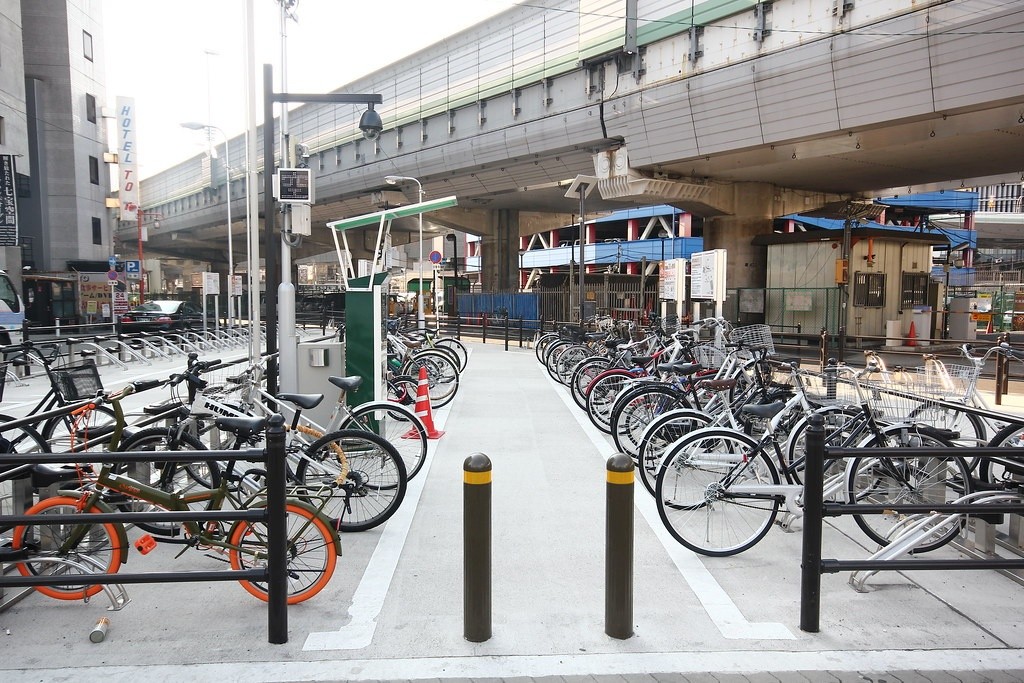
[632,331,644,341]
[859,364,979,423]
[814,372,857,407]
[661,313,682,335]
[207,362,250,408]
[616,325,630,341]
[51,359,104,405]
[700,340,726,369]
[728,324,776,359]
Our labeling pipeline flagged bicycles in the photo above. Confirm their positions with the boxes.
[536,311,1024,557]
[0,318,468,606]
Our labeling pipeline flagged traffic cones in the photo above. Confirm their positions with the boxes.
[401,367,445,440]
[986,318,994,332]
[907,320,919,346]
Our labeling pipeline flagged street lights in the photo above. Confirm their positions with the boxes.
[605,238,621,274]
[446,234,457,318]
[262,63,383,419]
[182,122,232,331]
[138,209,159,305]
[658,231,668,260]
[385,176,426,338]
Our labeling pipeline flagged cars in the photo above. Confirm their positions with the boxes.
[121,299,222,342]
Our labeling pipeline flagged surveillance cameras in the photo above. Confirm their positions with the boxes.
[359,108,383,141]
